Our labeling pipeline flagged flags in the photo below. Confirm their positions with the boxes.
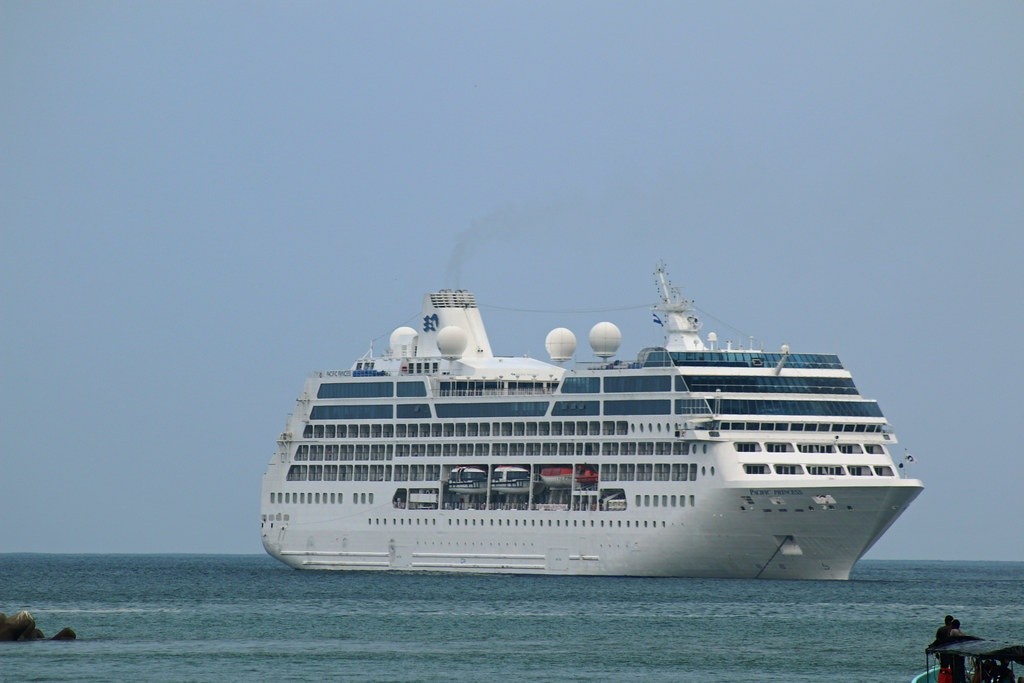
[653,313,666,327]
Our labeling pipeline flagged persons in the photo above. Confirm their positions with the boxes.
[946,618,967,683]
[935,615,953,671]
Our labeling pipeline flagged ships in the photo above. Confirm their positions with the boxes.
[260,263,927,582]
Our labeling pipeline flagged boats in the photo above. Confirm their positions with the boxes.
[538,467,595,493]
[448,466,486,494]
[491,466,544,496]
